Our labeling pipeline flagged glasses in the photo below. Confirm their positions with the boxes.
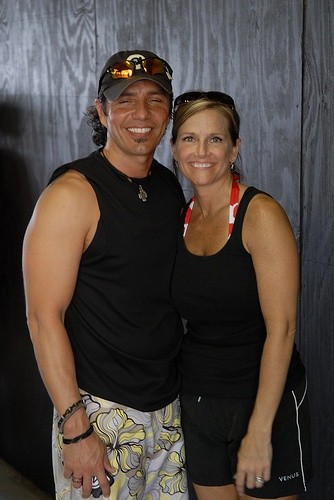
[173,91,236,112]
[98,57,173,94]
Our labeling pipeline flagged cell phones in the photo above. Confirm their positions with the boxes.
[62,461,115,497]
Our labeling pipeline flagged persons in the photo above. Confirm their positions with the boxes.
[169,90,315,500]
[22,50,188,500]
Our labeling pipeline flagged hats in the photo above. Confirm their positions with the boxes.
[98,50,173,100]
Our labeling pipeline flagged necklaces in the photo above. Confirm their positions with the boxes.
[100,148,147,205]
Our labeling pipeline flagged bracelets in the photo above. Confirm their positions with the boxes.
[58,399,86,433]
[63,426,93,445]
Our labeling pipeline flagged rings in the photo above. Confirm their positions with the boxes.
[72,479,82,482]
[256,476,264,484]
